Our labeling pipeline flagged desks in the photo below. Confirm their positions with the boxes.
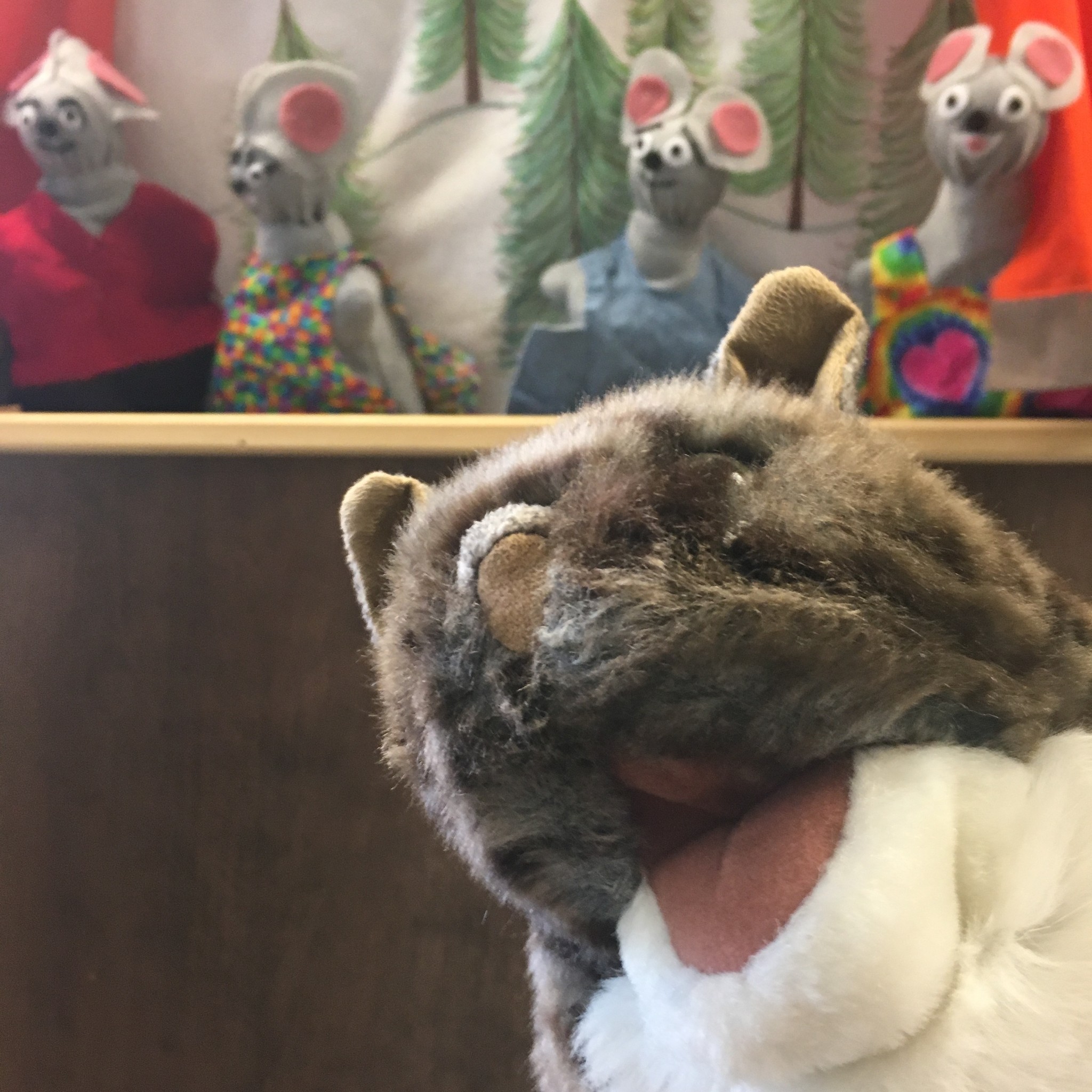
[1,408,1092,1092]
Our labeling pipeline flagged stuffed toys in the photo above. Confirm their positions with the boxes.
[852,22,1092,421]
[506,46,773,415]
[202,60,483,414]
[0,30,227,413]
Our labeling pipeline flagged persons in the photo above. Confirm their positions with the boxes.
[339,264,1092,1092]
